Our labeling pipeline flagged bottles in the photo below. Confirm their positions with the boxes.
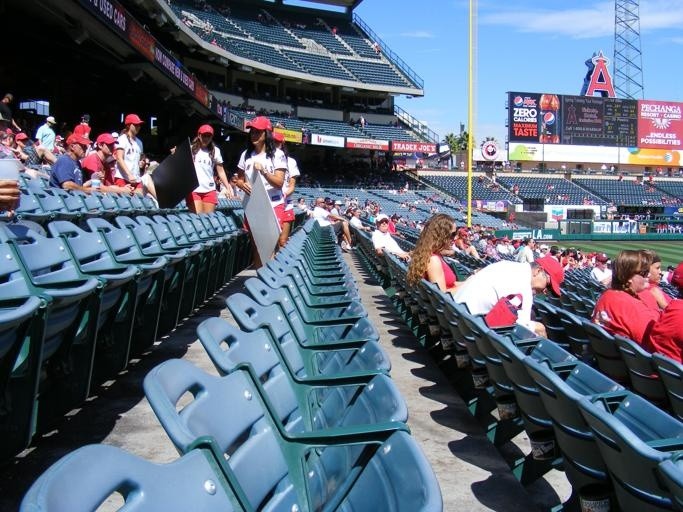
[91,172,100,191]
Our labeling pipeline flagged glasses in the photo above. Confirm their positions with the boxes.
[377,220,389,225]
[542,270,552,290]
[633,269,651,279]
[448,228,460,240]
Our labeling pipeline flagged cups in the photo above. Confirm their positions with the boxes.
[0,158,21,188]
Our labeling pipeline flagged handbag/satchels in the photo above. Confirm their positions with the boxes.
[484,294,524,329]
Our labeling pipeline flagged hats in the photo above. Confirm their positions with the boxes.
[534,256,564,298]
[596,253,610,261]
[375,213,391,223]
[0,93,285,149]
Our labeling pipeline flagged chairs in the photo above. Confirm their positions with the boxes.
[226,104,421,142]
[166,0,411,88]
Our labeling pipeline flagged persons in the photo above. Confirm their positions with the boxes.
[170,123,233,213]
[0,93,161,217]
[301,156,682,365]
[272,135,301,246]
[233,115,288,269]
[218,98,370,130]
